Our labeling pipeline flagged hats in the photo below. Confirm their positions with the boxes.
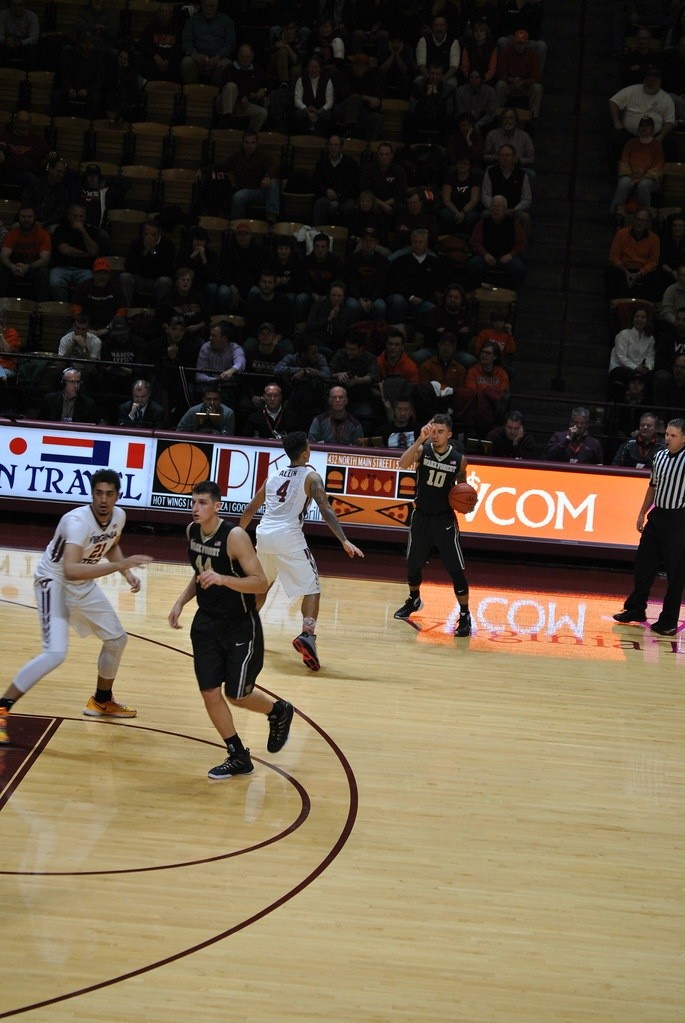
[627,371,644,383]
[513,29,528,43]
[90,258,111,271]
[639,116,654,127]
[84,164,101,175]
[235,221,252,233]
[258,323,275,334]
[111,317,129,335]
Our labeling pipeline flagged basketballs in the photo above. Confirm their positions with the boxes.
[448,483,478,514]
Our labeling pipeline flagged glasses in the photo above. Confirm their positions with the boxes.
[65,380,81,385]
[480,349,492,357]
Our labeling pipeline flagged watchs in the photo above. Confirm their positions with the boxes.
[81,348,88,356]
[105,325,112,331]
[263,87,268,92]
[151,251,158,255]
[0,333,3,337]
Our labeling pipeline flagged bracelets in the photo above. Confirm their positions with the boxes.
[460,208,468,216]
[285,46,291,50]
[123,570,131,577]
[408,295,415,302]
[304,369,311,377]
[565,434,573,441]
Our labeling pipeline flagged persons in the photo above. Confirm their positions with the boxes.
[0,0,685,473]
[394,413,479,638]
[0,469,152,745]
[613,419,685,633]
[169,482,293,780]
[238,432,364,672]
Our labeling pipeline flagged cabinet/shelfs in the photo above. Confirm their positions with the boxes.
[1,1,684,471]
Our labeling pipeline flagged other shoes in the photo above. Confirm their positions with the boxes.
[650,617,677,636]
[613,610,647,623]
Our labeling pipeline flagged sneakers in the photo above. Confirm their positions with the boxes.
[393,595,424,619]
[267,701,293,753]
[209,747,254,779]
[81,693,137,719]
[292,632,322,672]
[454,611,472,637]
[0,706,11,747]
